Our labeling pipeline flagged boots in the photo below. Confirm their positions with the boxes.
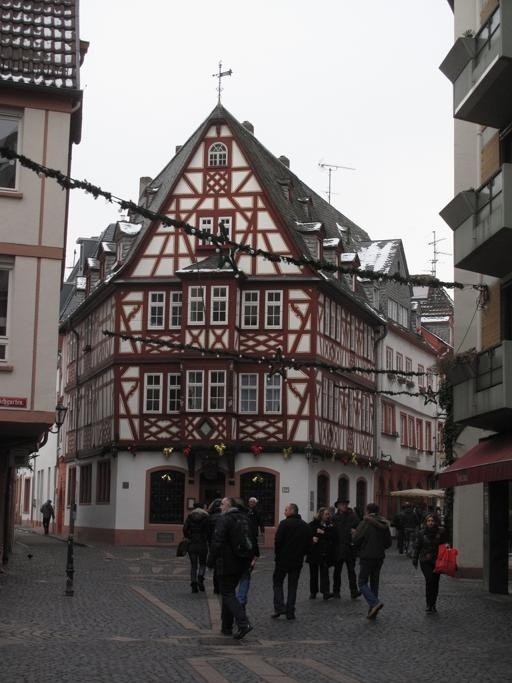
[190,576,206,593]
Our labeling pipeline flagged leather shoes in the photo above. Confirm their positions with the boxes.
[333,590,341,598]
[366,600,384,620]
[323,592,336,600]
[233,622,253,640]
[270,607,297,620]
[309,592,317,600]
[351,591,362,599]
[221,627,233,635]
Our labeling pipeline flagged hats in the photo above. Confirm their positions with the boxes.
[334,496,350,508]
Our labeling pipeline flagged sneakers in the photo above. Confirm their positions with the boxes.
[424,605,437,613]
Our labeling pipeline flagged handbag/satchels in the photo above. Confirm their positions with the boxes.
[432,543,459,577]
[176,540,189,557]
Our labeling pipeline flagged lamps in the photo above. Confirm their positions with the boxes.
[472,283,489,311]
[381,450,394,470]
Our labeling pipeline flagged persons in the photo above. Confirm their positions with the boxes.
[39,500,55,536]
[182,495,449,641]
